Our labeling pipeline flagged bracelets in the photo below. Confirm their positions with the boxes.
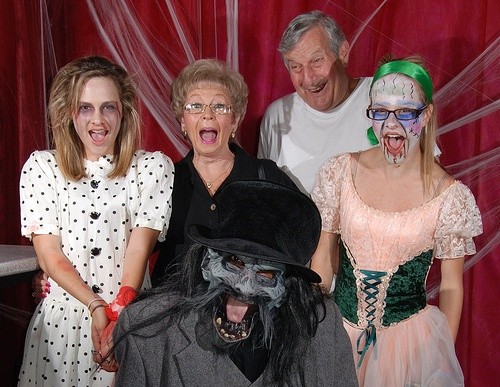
[86,296,110,317]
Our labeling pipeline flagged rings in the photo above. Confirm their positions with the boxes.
[32,292,35,297]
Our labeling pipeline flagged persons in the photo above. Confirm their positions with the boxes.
[257,9,443,302]
[18,55,175,387]
[308,59,482,387]
[31,58,322,301]
[111,179,362,386]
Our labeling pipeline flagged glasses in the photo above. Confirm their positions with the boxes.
[367,103,429,120]
[183,102,233,115]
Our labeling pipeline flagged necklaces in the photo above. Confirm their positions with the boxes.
[194,153,234,188]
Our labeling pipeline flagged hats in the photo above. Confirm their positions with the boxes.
[184,179,323,284]
